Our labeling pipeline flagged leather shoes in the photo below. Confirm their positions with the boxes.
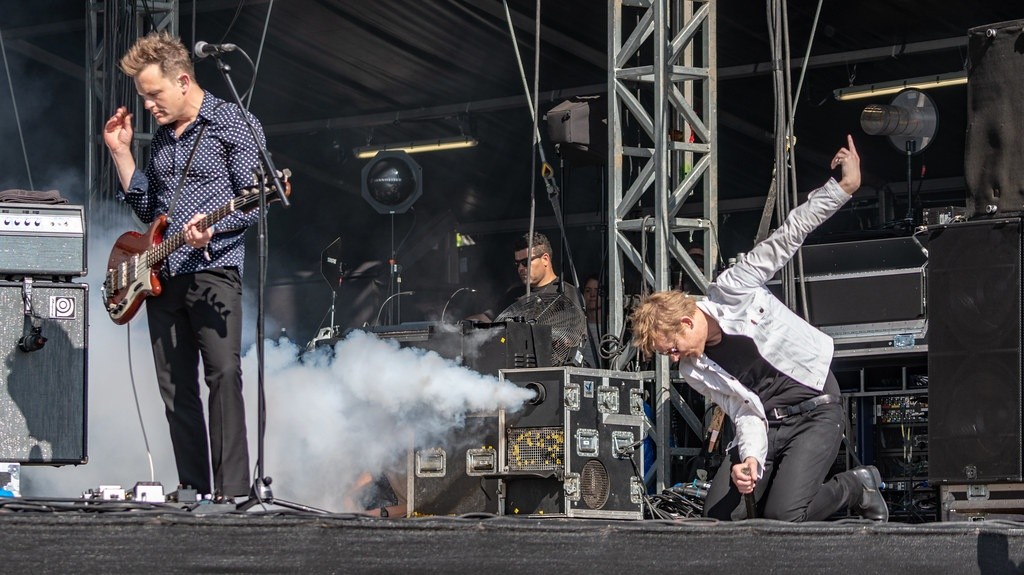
[850,464,889,524]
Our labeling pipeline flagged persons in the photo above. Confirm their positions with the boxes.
[581,274,609,365]
[101,29,276,510]
[626,133,890,525]
[463,231,588,362]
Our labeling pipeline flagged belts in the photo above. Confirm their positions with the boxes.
[766,394,843,420]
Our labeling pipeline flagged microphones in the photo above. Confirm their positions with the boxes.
[441,287,476,326]
[194,41,236,58]
[740,468,756,519]
[375,290,416,326]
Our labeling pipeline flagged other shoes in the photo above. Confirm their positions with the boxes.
[165,491,179,503]
[214,495,236,505]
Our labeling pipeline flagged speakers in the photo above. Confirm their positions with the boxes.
[0,281,88,465]
[927,218,1024,484]
[963,18,1024,219]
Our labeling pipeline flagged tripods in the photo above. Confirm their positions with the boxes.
[201,58,331,515]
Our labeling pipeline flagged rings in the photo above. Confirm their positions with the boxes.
[835,157,840,164]
[191,241,196,246]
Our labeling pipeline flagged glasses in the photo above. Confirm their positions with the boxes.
[658,331,679,356]
[511,254,543,269]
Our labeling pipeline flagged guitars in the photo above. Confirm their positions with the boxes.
[101,168,292,325]
